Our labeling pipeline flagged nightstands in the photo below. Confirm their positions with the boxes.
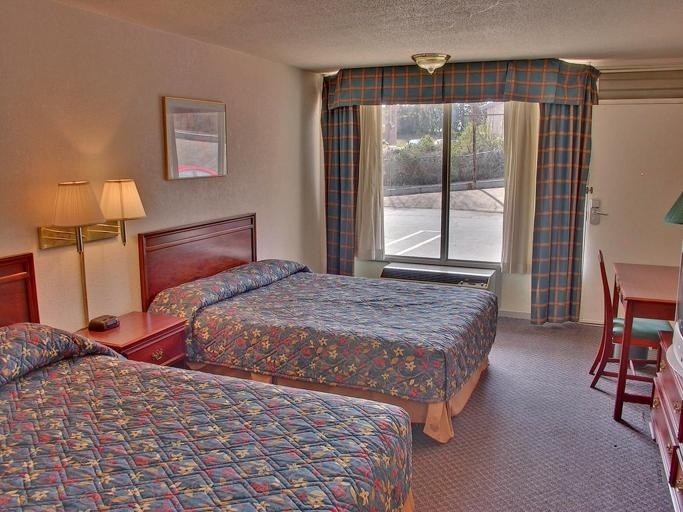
[71,310,187,370]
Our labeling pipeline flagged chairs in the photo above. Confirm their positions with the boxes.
[589,249,674,412]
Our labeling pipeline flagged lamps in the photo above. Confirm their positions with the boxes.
[37,177,147,255]
[410,53,452,76]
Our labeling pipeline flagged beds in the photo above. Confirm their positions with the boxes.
[134,212,496,430]
[0,252,412,511]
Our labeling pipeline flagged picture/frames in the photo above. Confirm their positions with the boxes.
[161,96,229,181]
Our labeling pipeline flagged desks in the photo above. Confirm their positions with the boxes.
[611,263,680,423]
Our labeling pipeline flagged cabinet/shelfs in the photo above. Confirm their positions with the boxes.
[647,327,682,511]
